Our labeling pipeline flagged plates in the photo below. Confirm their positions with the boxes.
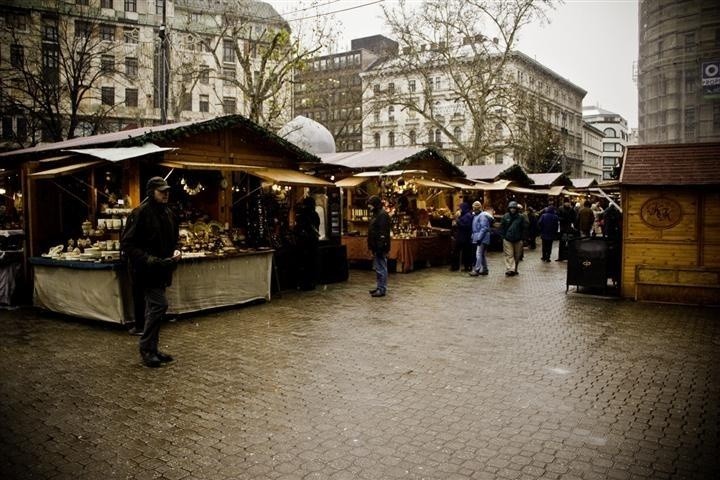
[193,222,212,242]
[208,221,225,235]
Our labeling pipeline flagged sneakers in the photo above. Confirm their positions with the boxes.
[504,270,520,277]
[139,348,174,367]
[129,325,144,336]
[540,257,552,262]
[448,266,489,276]
[369,288,387,298]
[555,256,570,262]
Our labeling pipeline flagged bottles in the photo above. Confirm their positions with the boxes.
[390,215,432,239]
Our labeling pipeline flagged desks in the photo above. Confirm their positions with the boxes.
[344,230,458,274]
[25,236,279,334]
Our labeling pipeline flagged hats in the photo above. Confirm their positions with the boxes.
[146,176,171,192]
[472,201,482,209]
[508,201,517,208]
[366,195,380,205]
[459,203,470,211]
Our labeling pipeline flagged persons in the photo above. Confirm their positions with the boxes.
[127,257,143,336]
[296,196,320,291]
[449,198,620,277]
[123,176,182,367]
[366,195,391,297]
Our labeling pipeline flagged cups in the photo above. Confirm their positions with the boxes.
[351,208,368,222]
[179,232,225,257]
[42,208,134,263]
